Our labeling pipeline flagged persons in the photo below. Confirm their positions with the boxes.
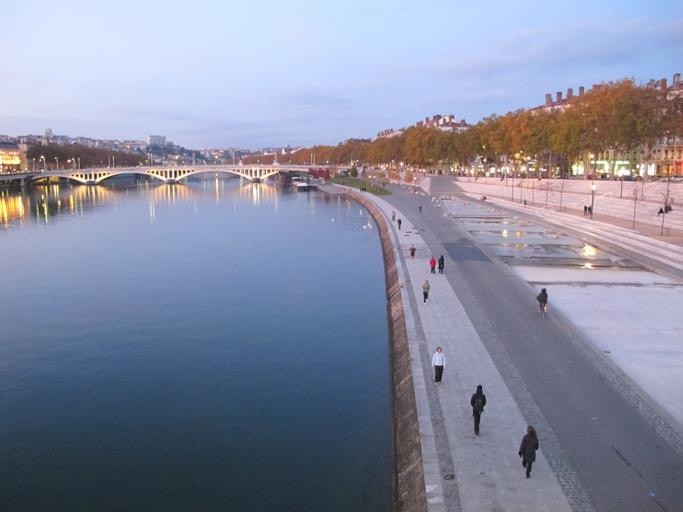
[518,425,539,479]
[536,288,548,313]
[409,244,417,264]
[417,205,423,212]
[583,205,592,216]
[397,219,401,229]
[421,280,431,305]
[429,254,444,274]
[432,346,446,385]
[391,211,396,221]
[523,199,527,207]
[471,385,486,435]
[657,204,672,216]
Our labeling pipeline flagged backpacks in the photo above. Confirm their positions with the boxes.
[474,399,483,412]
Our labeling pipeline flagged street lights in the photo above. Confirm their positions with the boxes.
[591,185,597,218]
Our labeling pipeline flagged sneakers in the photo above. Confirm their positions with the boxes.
[434,382,441,385]
